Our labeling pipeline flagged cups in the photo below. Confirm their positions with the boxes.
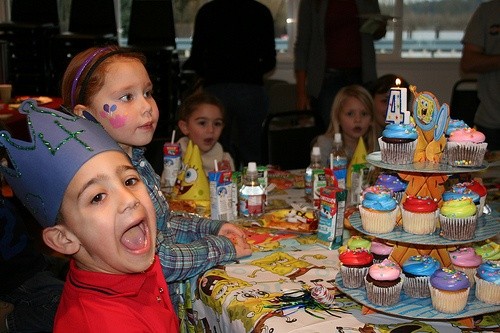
[0,85,12,100]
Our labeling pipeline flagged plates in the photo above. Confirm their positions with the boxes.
[33,97,52,105]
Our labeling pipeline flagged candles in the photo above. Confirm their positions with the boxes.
[385,78,410,124]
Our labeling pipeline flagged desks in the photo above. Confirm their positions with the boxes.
[177,169,500,333]
[0,95,63,141]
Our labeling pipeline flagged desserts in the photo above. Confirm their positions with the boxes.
[337,236,500,314]
[378,118,488,167]
[358,173,486,240]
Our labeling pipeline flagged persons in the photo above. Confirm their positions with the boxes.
[0,0,500,310]
[0,100,181,333]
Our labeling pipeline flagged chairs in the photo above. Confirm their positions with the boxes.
[450,79,481,126]
[262,109,320,169]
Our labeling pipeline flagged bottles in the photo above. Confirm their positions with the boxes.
[304,146,325,201]
[326,133,347,170]
[238,162,266,219]
